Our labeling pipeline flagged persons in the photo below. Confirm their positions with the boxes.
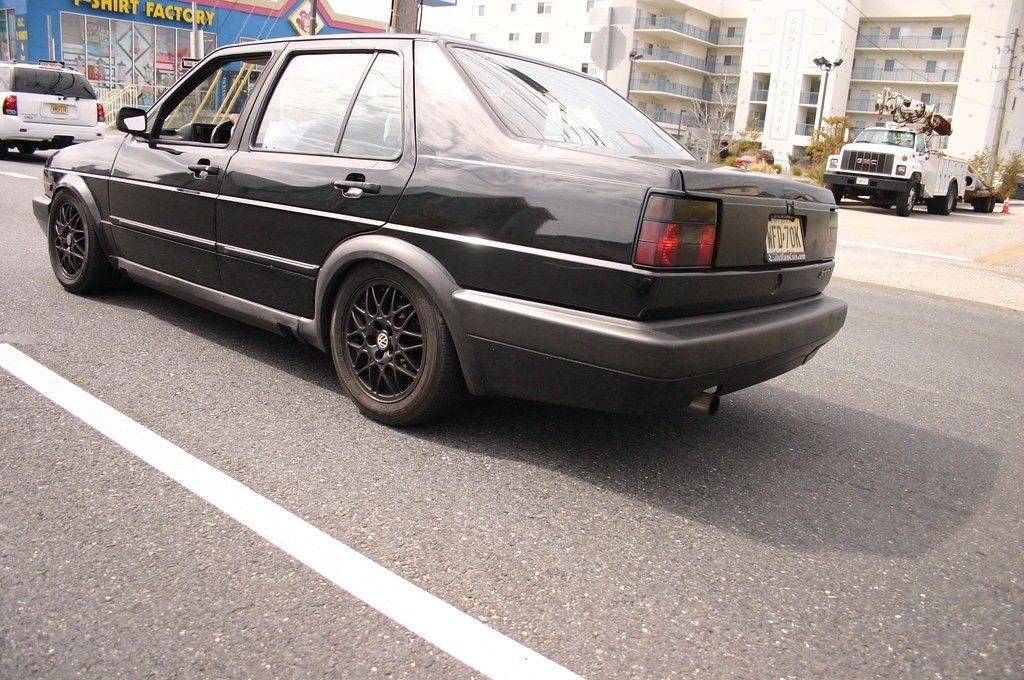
[230,114,239,136]
[721,141,729,160]
